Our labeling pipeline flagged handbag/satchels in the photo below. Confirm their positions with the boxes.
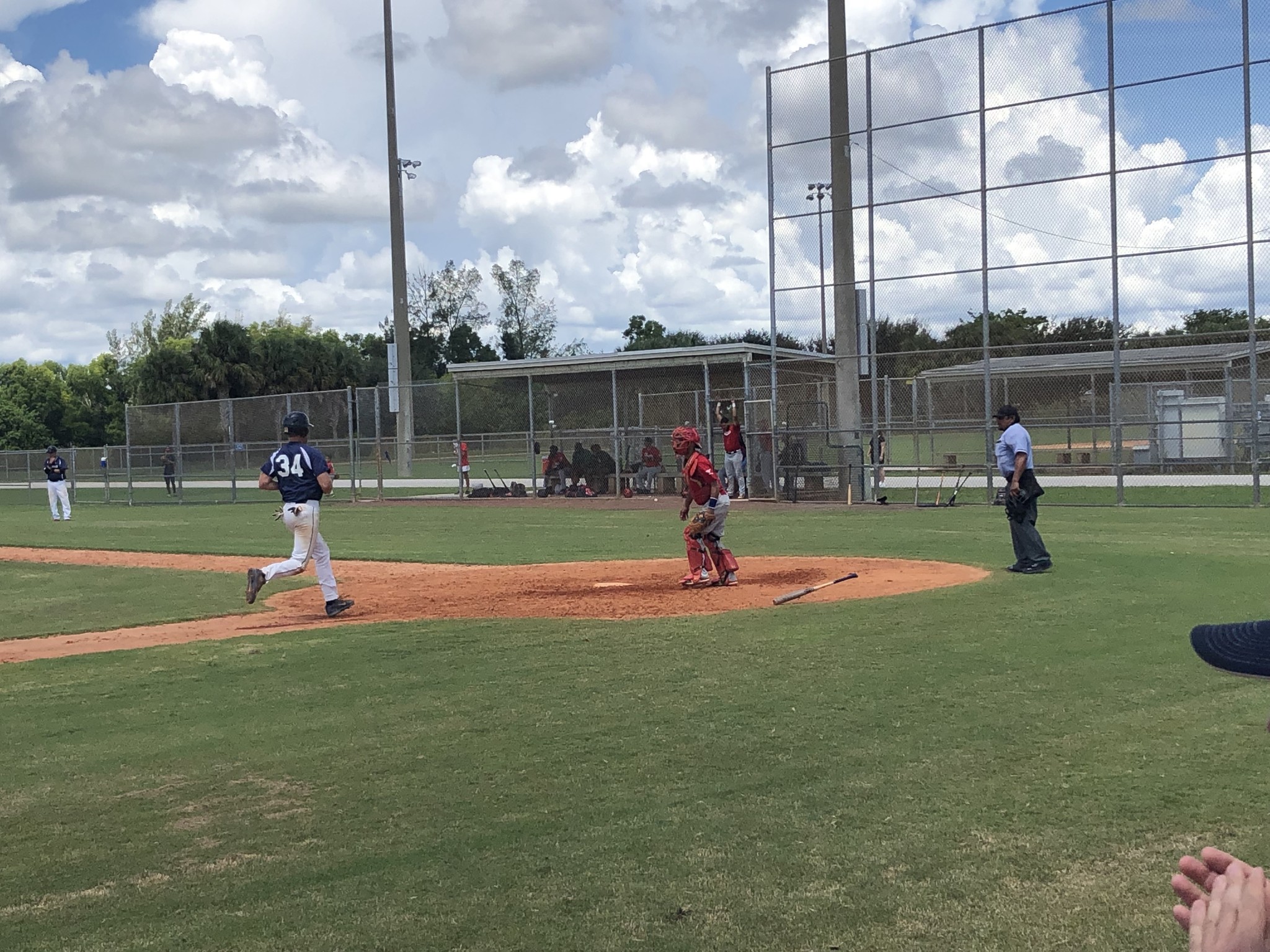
[510,481,598,497]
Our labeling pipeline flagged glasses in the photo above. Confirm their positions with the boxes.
[997,416,1006,419]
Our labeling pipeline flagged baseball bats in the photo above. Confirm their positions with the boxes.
[847,464,852,504]
[494,469,508,488]
[483,469,497,489]
[773,572,858,605]
[914,475,919,505]
[945,472,972,507]
[936,471,944,505]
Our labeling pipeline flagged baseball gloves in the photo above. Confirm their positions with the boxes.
[689,511,715,533]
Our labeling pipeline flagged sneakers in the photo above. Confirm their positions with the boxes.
[1007,562,1026,572]
[245,567,266,604]
[325,600,354,618]
[1023,559,1053,574]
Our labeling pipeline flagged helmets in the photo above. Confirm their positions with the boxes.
[623,487,633,497]
[45,445,57,453]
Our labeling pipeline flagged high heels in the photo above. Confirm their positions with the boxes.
[167,493,172,497]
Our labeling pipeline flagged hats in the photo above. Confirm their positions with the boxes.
[992,406,1019,417]
[671,426,703,449]
[283,411,314,428]
[325,453,332,459]
[1188,618,1270,681]
[165,446,171,451]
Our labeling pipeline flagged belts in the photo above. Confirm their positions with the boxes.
[726,451,737,455]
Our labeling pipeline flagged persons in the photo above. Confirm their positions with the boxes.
[245,411,355,617]
[715,397,779,498]
[635,437,662,494]
[43,445,71,521]
[324,454,335,498]
[991,405,1054,574]
[867,429,886,487]
[544,443,617,495]
[160,446,177,497]
[454,435,470,495]
[670,425,740,587]
[1169,620,1270,952]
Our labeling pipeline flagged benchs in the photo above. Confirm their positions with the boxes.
[942,450,998,466]
[1055,447,1094,464]
[533,470,839,494]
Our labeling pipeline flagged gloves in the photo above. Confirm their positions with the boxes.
[287,506,302,516]
[272,506,284,520]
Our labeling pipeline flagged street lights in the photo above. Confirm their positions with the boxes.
[805,183,833,443]
[390,159,422,478]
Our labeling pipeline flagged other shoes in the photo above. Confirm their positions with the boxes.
[711,577,738,585]
[872,480,886,486]
[465,490,470,494]
[324,494,335,498]
[738,496,744,499]
[683,575,710,584]
[173,493,177,497]
[454,490,464,495]
[729,496,734,498]
